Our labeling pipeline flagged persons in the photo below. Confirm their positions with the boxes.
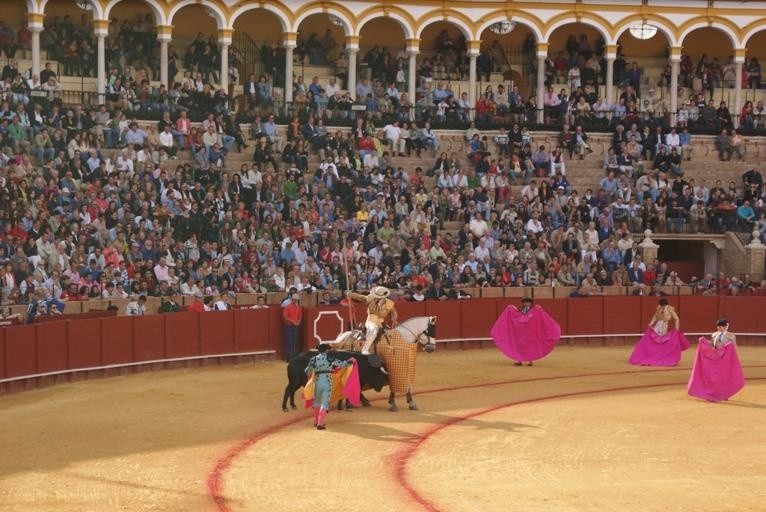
[510,297,536,367]
[344,285,399,357]
[696,317,737,402]
[646,297,681,337]
[337,290,357,331]
[280,293,304,363]
[302,342,358,432]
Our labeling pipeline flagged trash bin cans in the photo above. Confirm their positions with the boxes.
[102,128,112,147]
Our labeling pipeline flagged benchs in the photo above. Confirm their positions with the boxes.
[119,56,161,94]
[269,87,284,100]
[477,64,523,103]
[382,133,473,193]
[292,62,348,97]
[0,48,98,110]
[208,68,244,99]
[551,76,571,102]
[681,135,766,198]
[758,60,766,84]
[430,81,470,102]
[484,133,615,199]
[597,85,606,99]
[613,56,670,117]
[678,87,766,122]
[462,282,694,299]
[163,292,289,306]
[62,297,163,316]
[4,305,29,319]
[173,49,188,84]
[297,290,342,307]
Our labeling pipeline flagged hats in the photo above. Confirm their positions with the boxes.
[717,319,727,326]
[292,293,301,300]
[659,298,668,303]
[369,285,390,299]
[315,344,332,350]
[344,290,352,296]
[520,296,533,303]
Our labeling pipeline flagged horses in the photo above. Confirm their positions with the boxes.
[334,315,438,412]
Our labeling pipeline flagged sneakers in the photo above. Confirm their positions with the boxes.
[313,423,326,429]
[513,361,535,366]
[360,350,372,355]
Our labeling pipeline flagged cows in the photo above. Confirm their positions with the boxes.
[279,349,390,412]
